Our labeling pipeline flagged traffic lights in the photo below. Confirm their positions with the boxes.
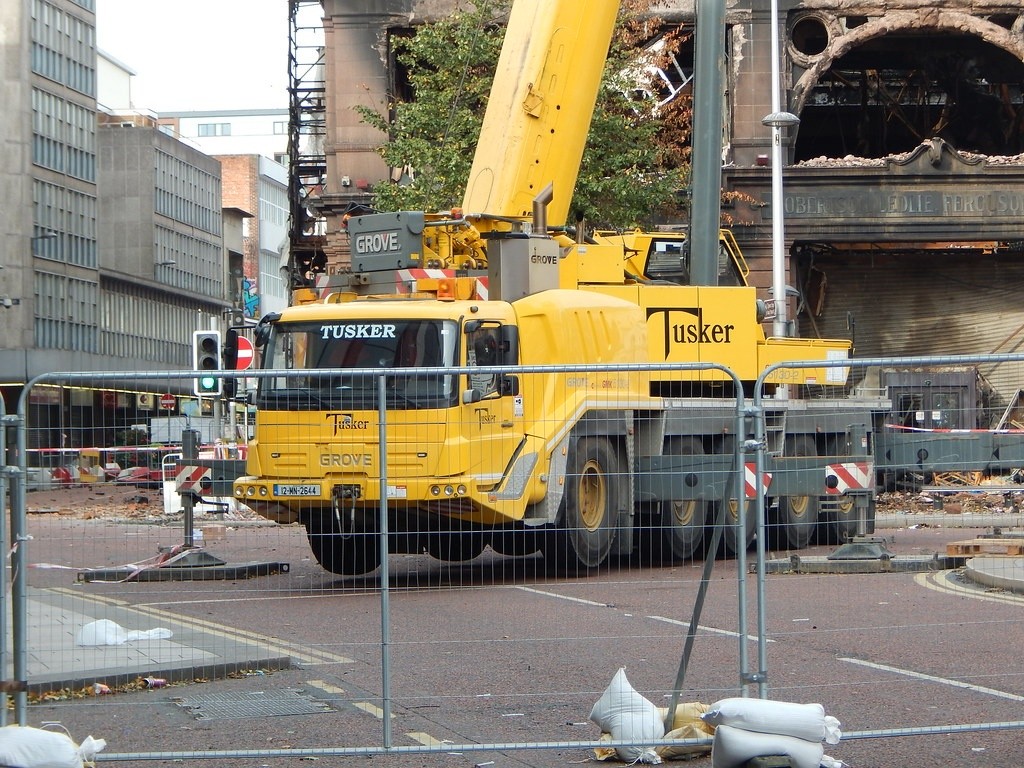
[191,331,223,396]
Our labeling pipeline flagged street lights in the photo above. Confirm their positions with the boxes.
[764,114,800,341]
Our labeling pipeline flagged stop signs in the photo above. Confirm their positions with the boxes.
[234,337,255,370]
[161,393,175,410]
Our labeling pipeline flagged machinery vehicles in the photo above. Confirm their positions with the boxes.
[155,2,1024,571]
